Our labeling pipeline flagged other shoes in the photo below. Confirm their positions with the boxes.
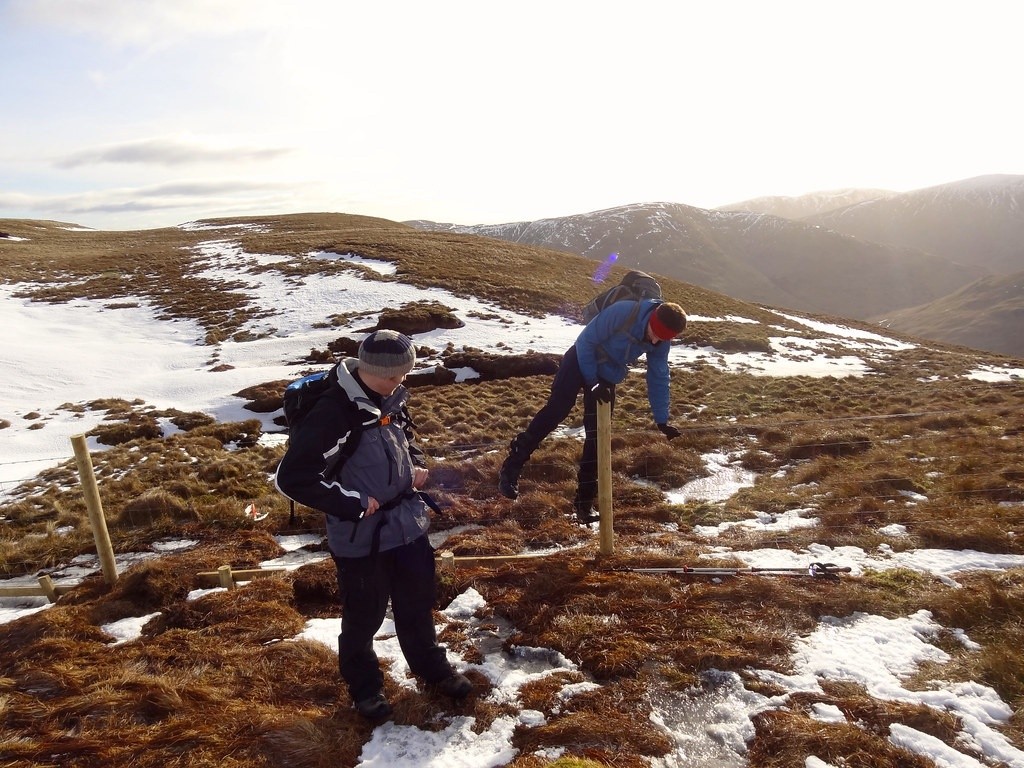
[578,508,600,522]
[498,464,519,500]
[355,691,390,717]
[433,666,473,695]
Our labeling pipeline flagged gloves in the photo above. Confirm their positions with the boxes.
[657,423,681,441]
[589,382,616,405]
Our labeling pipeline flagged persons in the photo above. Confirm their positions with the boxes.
[498,299,688,524]
[272,328,475,719]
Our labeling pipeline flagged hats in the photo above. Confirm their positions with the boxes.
[358,329,416,377]
[650,302,687,340]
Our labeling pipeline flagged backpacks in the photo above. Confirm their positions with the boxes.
[581,271,661,337]
[284,372,364,481]
